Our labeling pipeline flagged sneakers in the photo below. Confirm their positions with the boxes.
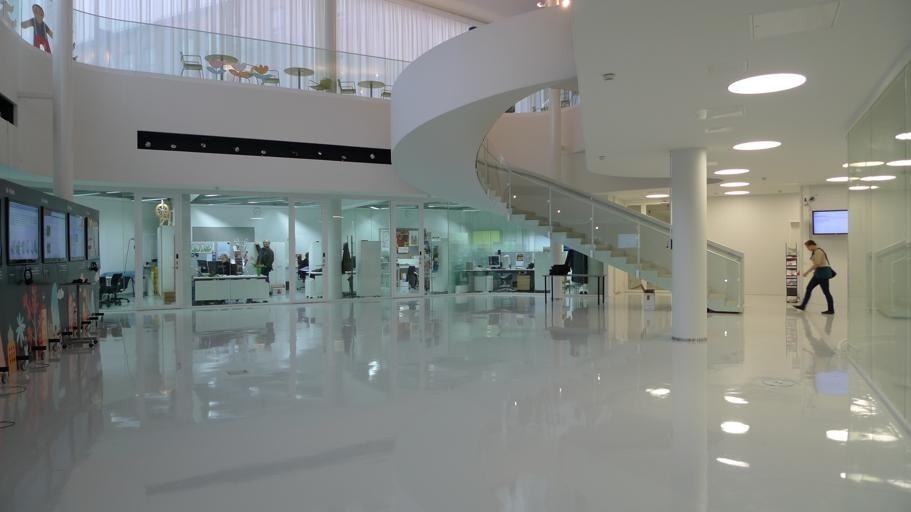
[822,310,834,314]
[794,305,805,310]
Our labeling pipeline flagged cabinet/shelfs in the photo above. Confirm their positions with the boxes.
[787,240,797,299]
[157,225,176,295]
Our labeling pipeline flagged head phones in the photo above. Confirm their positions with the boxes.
[24,269,33,285]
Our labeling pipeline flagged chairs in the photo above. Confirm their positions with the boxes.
[99,273,130,307]
[179,50,393,100]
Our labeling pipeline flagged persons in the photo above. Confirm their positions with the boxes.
[254,239,275,283]
[792,239,836,314]
[22,4,53,54]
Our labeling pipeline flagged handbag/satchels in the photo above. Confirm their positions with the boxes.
[813,265,837,281]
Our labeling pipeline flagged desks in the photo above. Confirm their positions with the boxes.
[542,273,607,306]
[60,281,97,348]
[193,275,269,304]
[454,268,534,292]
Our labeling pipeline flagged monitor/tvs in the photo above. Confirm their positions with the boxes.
[488,255,500,269]
[42,205,70,264]
[208,261,224,278]
[4,196,40,266]
[197,260,208,273]
[812,210,848,235]
[86,217,100,260]
[68,212,86,261]
